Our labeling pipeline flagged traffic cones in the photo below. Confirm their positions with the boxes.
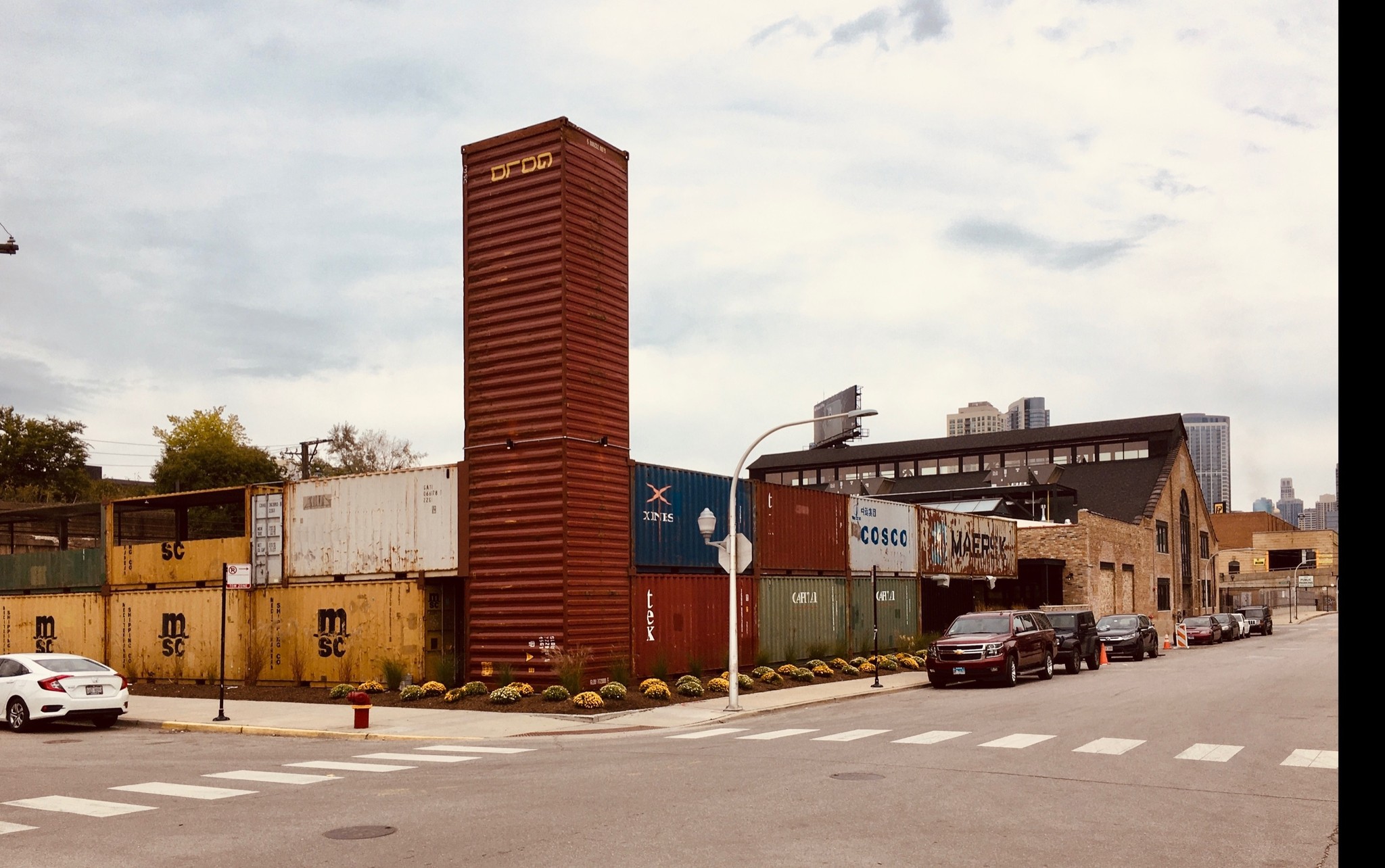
[1162,633,1172,650]
[1099,642,1111,665]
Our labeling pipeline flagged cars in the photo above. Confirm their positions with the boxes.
[1230,613,1251,639]
[1173,616,1222,645]
[0,653,129,734]
[1211,614,1240,642]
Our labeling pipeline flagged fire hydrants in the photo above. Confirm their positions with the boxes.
[346,692,373,729]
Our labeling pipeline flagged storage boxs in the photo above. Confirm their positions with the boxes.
[0,116,1017,693]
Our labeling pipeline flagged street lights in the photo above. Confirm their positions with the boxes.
[698,410,878,711]
[1206,548,1255,614]
[1294,559,1331,618]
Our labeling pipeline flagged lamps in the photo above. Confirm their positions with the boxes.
[507,438,514,450]
[601,436,607,446]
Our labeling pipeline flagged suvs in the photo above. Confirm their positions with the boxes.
[926,609,1059,689]
[1236,605,1273,635]
[1045,611,1101,674]
[1096,613,1159,661]
